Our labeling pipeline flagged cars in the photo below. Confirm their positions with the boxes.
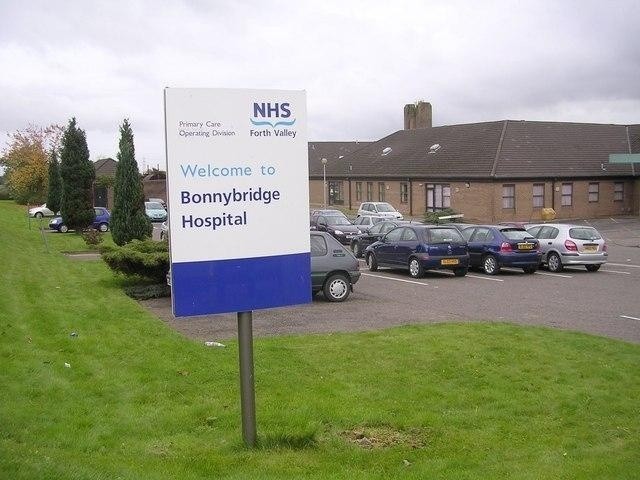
[48,207,110,233]
[143,198,169,241]
[166,231,360,302]
[29,203,61,219]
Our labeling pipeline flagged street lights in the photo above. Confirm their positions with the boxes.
[321,158,328,209]
